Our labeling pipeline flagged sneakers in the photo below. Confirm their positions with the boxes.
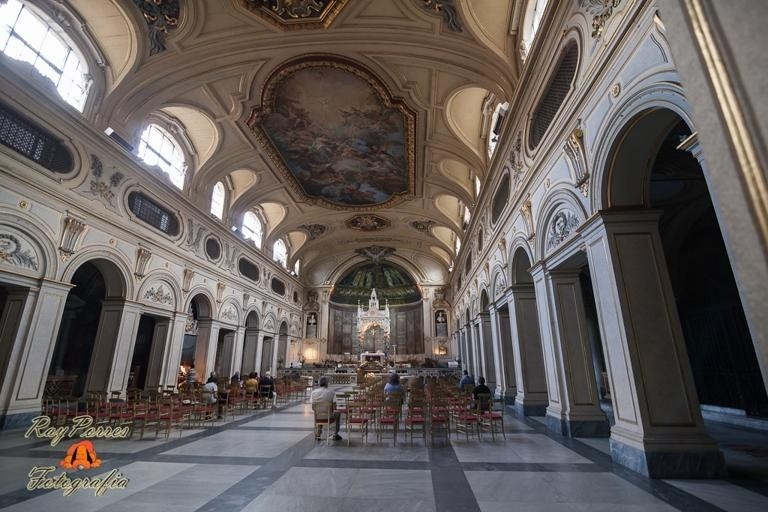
[333,435,342,441]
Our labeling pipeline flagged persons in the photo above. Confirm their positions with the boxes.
[383,373,404,411]
[259,370,277,409]
[244,371,259,409]
[230,371,240,386]
[310,376,342,442]
[207,371,218,383]
[201,375,225,422]
[467,376,491,427]
[288,362,294,368]
[436,312,446,323]
[308,314,316,324]
[185,363,196,390]
[458,369,474,396]
